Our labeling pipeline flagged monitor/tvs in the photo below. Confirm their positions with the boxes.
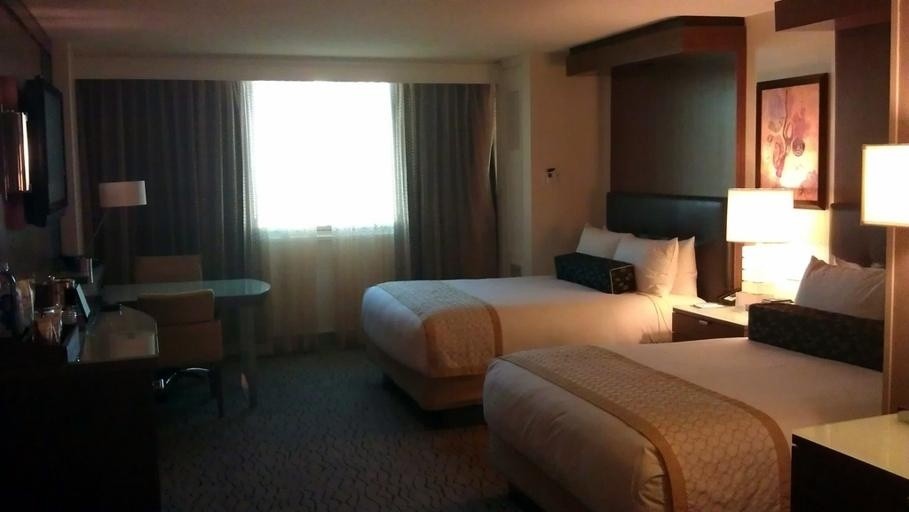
[23,74,68,218]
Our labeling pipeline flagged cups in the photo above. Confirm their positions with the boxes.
[36,305,63,344]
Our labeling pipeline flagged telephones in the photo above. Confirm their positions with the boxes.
[716,287,742,306]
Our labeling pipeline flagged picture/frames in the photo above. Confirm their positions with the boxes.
[755,73,830,211]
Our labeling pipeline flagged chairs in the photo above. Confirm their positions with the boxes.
[133,254,203,283]
[136,288,225,419]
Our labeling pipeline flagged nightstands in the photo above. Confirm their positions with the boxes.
[671,306,748,342]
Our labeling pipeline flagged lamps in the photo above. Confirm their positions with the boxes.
[725,187,794,309]
[87,180,148,240]
[861,144,908,227]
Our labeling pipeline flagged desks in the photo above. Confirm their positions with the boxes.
[0,304,161,512]
[101,278,271,409]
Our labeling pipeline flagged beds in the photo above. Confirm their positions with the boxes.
[483,202,886,512]
[358,191,735,423]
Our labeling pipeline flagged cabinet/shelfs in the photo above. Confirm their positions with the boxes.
[790,411,909,512]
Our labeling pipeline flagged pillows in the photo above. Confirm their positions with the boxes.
[748,252,883,372]
[554,222,697,295]
